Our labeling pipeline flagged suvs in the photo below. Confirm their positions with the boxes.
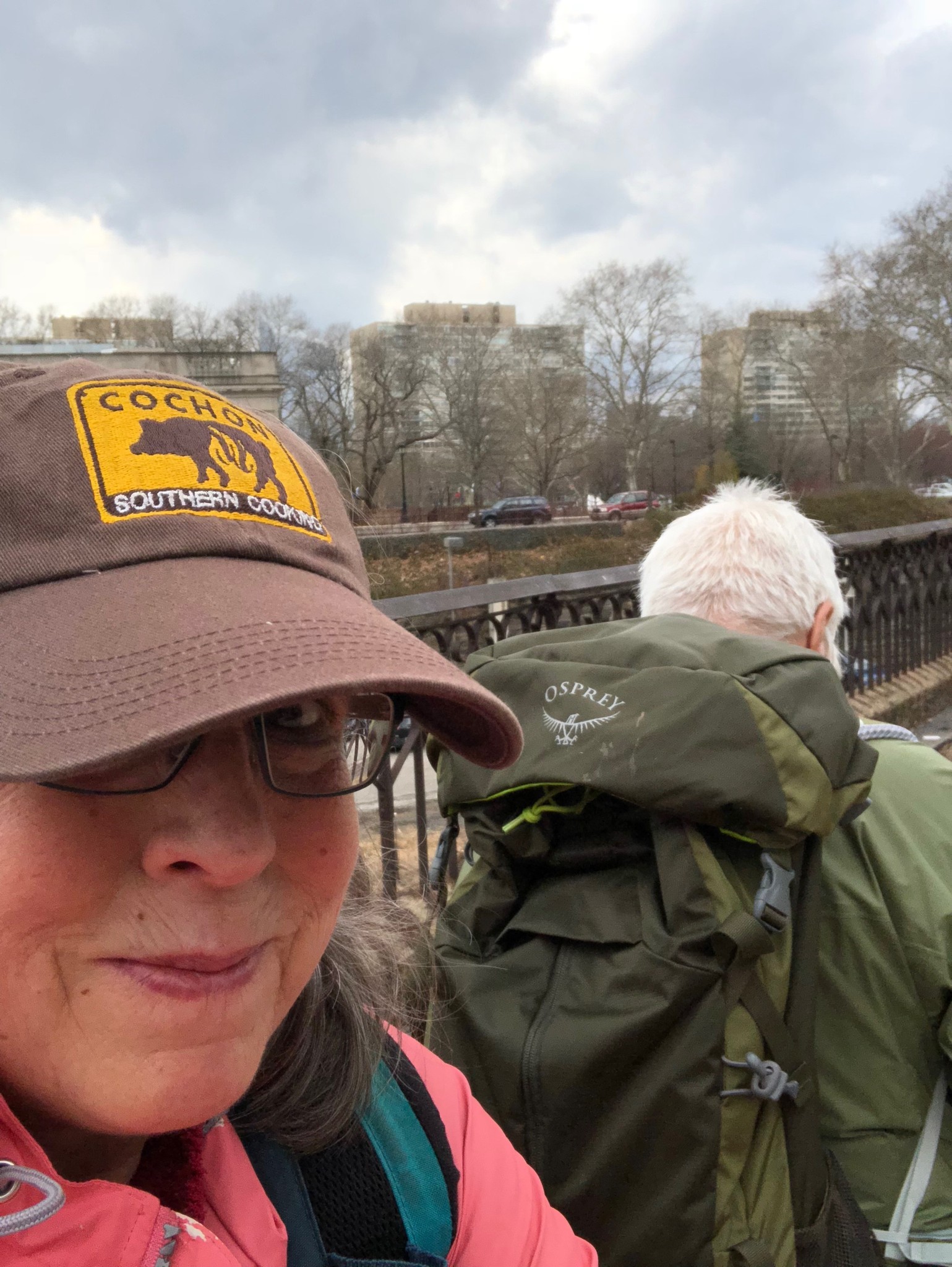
[468,495,553,528]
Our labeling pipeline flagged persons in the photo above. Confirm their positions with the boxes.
[1,354,597,1267]
[421,475,951,1267]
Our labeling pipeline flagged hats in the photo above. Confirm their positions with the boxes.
[0,362,525,778]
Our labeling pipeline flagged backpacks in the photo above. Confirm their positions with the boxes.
[425,615,948,1266]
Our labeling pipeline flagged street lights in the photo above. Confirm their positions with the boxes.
[397,442,410,523]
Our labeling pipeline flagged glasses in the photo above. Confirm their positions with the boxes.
[37,692,404,797]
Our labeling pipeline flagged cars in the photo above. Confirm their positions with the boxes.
[913,482,952,498]
[590,490,662,522]
